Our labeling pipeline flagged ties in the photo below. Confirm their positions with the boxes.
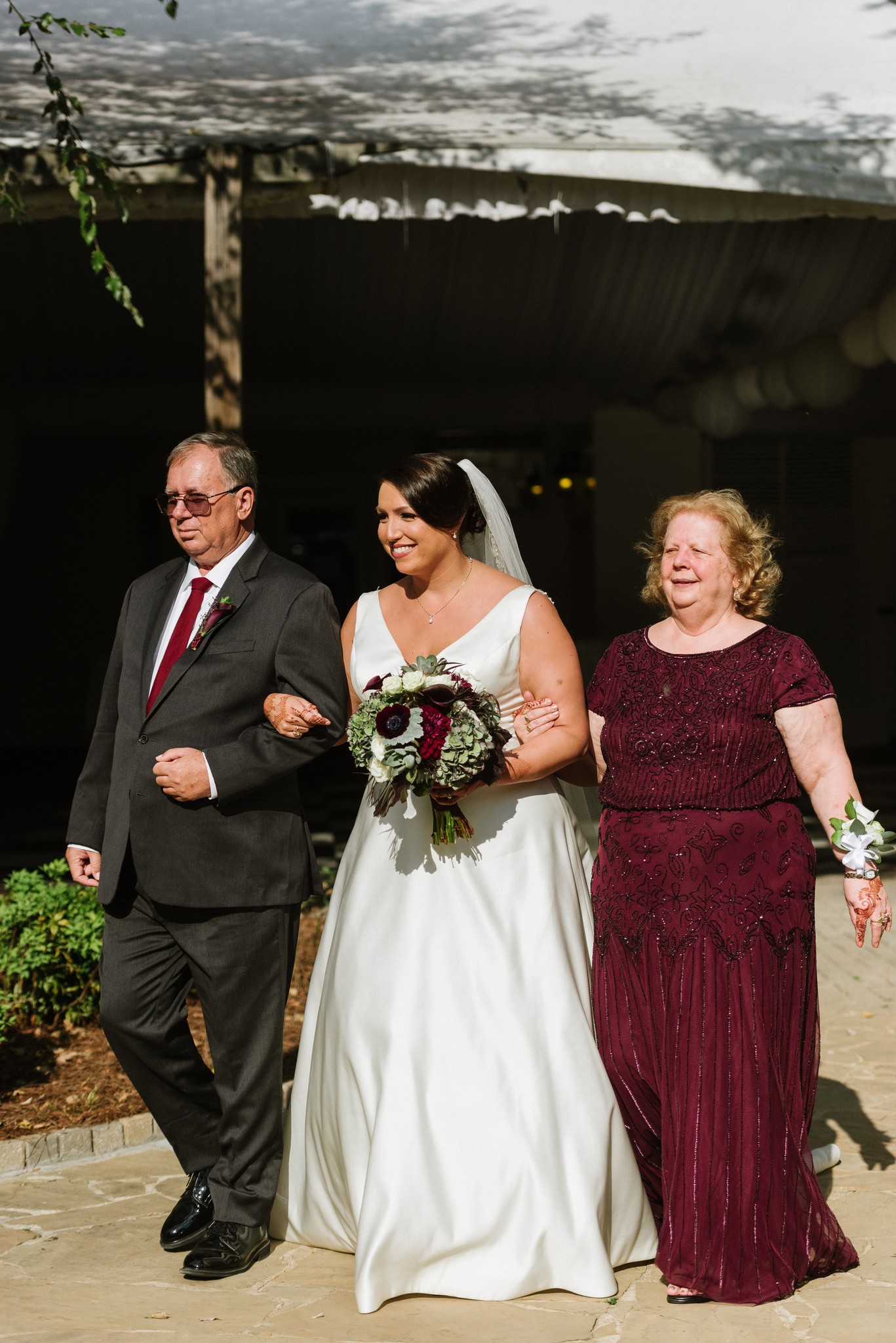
[145,576,215,719]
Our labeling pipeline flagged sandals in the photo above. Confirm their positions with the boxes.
[667,1294,709,1303]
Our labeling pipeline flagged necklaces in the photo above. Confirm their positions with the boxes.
[412,556,472,623]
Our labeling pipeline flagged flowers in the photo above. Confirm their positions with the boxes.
[192,596,237,651]
[347,653,513,846]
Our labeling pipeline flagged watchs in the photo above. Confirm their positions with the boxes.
[844,869,879,880]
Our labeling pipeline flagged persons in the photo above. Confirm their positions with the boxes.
[65,433,349,1278]
[516,490,892,1305]
[264,452,656,1313]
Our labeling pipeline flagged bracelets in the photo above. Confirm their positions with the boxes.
[828,791,896,876]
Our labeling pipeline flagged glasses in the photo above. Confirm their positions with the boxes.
[155,489,234,516]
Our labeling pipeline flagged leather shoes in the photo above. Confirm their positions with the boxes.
[159,1167,216,1249]
[179,1221,271,1276]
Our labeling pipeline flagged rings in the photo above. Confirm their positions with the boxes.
[448,791,452,799]
[524,713,530,723]
[526,723,532,733]
[871,916,885,923]
[887,913,892,917]
[293,726,300,737]
[880,913,887,917]
